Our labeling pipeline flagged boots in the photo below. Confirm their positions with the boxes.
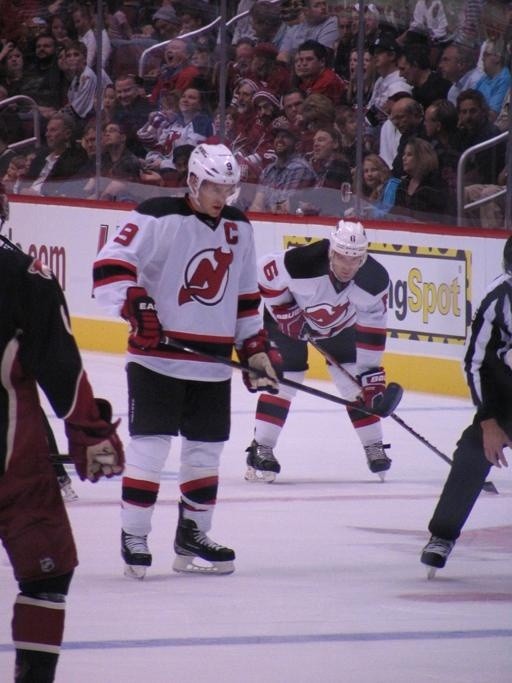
[363,440,394,484]
[169,515,237,577]
[118,524,153,582]
[419,533,457,585]
[243,438,282,486]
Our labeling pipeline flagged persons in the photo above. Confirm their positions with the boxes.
[421,234,512,568]
[93,143,278,567]
[0,0,512,230]
[245,223,404,482]
[0,187,125,682]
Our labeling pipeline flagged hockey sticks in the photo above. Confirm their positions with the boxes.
[160,334,404,419]
[308,335,501,494]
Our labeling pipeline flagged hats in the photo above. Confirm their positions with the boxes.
[267,115,302,143]
[365,32,404,57]
[236,76,272,95]
[251,87,283,111]
[149,3,182,27]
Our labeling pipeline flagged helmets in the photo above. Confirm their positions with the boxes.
[328,218,370,285]
[185,143,242,211]
